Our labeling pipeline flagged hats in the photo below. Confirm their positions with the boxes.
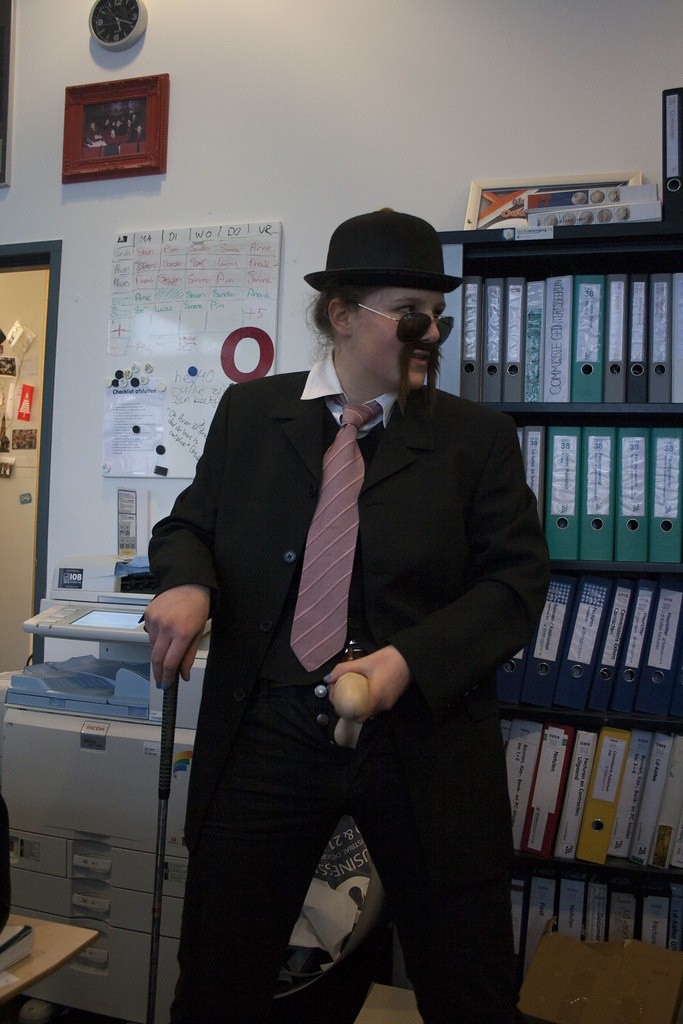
[302,208,465,293]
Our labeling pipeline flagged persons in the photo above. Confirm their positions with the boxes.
[143,212,551,1023]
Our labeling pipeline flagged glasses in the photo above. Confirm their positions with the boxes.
[352,303,455,347]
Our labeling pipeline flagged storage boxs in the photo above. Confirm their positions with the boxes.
[517,916,683,1024]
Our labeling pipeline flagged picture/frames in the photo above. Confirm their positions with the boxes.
[61,73,170,184]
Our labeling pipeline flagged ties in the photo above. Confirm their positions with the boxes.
[289,394,384,673]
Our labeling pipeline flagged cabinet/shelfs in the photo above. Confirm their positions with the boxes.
[437,220,682,881]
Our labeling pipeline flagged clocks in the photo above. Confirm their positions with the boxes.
[89,0,148,52]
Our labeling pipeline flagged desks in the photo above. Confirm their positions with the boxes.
[0,915,100,1008]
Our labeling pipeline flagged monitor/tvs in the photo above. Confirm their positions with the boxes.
[70,610,147,630]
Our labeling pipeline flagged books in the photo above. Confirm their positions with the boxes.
[0,924,40,974]
